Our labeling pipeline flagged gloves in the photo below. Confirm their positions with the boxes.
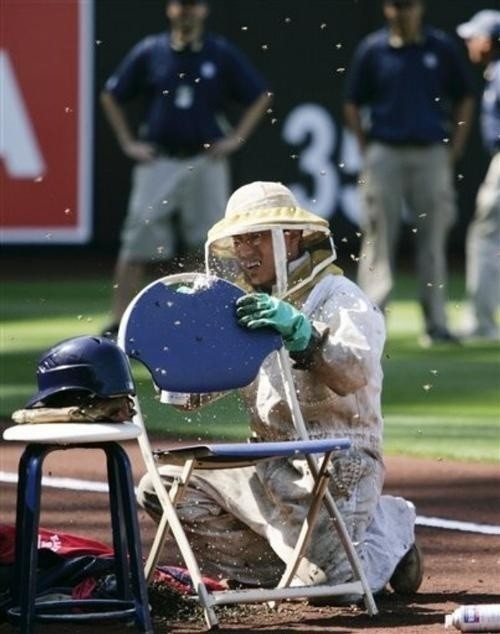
[234,290,314,352]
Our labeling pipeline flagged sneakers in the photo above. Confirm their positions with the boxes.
[388,546,422,596]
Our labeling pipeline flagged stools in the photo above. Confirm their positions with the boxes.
[2,416,160,632]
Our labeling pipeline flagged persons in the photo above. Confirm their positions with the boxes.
[343,1,477,342]
[134,180,425,601]
[100,0,274,343]
[456,8,500,342]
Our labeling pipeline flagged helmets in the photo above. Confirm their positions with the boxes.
[25,336,137,407]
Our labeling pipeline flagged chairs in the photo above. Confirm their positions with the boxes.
[120,261,380,630]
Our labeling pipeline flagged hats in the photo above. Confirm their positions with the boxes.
[206,180,329,257]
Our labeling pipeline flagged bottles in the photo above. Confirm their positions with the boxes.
[159,283,198,407]
[444,603,500,631]
